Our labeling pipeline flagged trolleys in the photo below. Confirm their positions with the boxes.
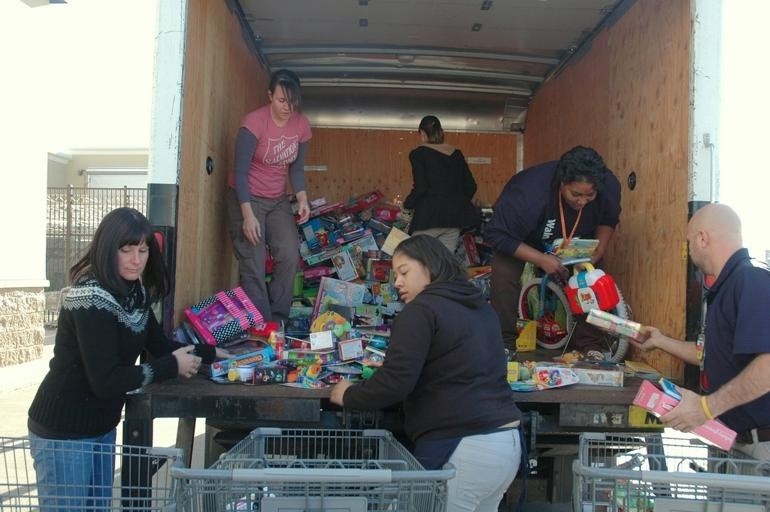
[0,434,188,512]
[168,425,460,512]
[568,429,770,512]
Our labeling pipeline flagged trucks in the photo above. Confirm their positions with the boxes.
[136,0,727,442]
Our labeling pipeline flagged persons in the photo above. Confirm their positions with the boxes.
[27,207,237,511]
[399,114,477,256]
[481,146,622,365]
[626,203,770,505]
[327,235,531,512]
[226,68,312,332]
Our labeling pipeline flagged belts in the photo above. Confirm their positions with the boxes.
[736,427,769,444]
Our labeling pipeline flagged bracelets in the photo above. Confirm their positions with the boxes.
[701,395,714,419]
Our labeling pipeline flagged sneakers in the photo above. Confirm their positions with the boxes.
[505,346,518,362]
[580,352,605,366]
